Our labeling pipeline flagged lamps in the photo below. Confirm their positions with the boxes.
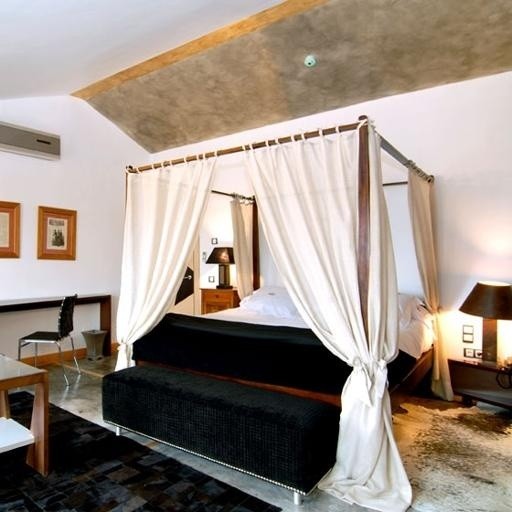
[459,281,512,361]
[205,247,237,290]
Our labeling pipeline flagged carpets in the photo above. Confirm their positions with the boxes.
[0,388,282,512]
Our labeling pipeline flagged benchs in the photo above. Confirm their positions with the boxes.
[102,364,342,506]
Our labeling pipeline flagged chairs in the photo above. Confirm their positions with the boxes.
[16,293,82,388]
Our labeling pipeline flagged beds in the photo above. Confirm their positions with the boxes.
[131,281,436,420]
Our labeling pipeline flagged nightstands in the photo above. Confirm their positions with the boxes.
[447,353,512,424]
[200,287,239,315]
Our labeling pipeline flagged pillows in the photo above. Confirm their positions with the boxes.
[239,282,419,330]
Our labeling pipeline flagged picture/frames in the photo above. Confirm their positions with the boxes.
[0,200,77,263]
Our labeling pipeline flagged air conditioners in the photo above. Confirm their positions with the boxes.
[0,120,62,163]
